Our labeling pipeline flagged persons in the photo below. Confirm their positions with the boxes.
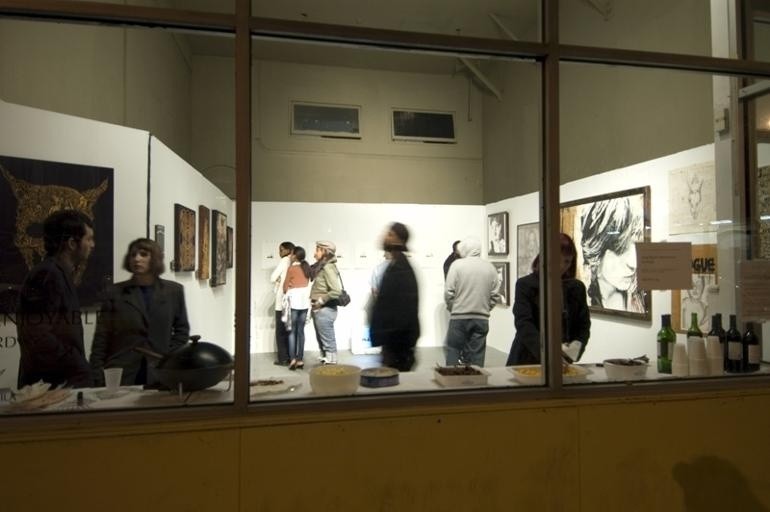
[89,239,191,392]
[443,240,462,280]
[270,242,296,367]
[506,233,590,366]
[370,221,420,371]
[16,208,93,387]
[582,197,646,314]
[282,246,313,370]
[682,274,709,332]
[441,236,501,368]
[488,216,504,252]
[518,229,538,277]
[497,266,504,301]
[363,250,392,342]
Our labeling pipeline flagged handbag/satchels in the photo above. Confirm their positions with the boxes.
[322,262,351,307]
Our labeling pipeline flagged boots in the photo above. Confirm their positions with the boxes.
[317,350,338,364]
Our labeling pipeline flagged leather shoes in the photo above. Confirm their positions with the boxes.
[274,359,290,366]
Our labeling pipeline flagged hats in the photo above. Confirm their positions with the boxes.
[315,240,337,256]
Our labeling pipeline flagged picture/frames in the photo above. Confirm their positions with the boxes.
[197,204,212,280]
[486,211,511,256]
[226,226,235,270]
[172,203,198,273]
[490,261,511,308]
[558,183,654,323]
[516,221,540,282]
[210,209,229,288]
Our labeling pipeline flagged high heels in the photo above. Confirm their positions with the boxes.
[287,358,296,370]
[295,359,305,369]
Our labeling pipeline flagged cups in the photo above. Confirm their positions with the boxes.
[669,334,725,378]
[103,368,123,393]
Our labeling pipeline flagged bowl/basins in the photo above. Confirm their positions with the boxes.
[358,368,402,387]
[508,365,595,384]
[309,363,361,394]
[603,359,648,381]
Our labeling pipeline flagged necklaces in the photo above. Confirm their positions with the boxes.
[309,239,343,364]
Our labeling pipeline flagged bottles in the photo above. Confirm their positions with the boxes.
[710,312,762,374]
[654,313,678,374]
[687,311,704,339]
